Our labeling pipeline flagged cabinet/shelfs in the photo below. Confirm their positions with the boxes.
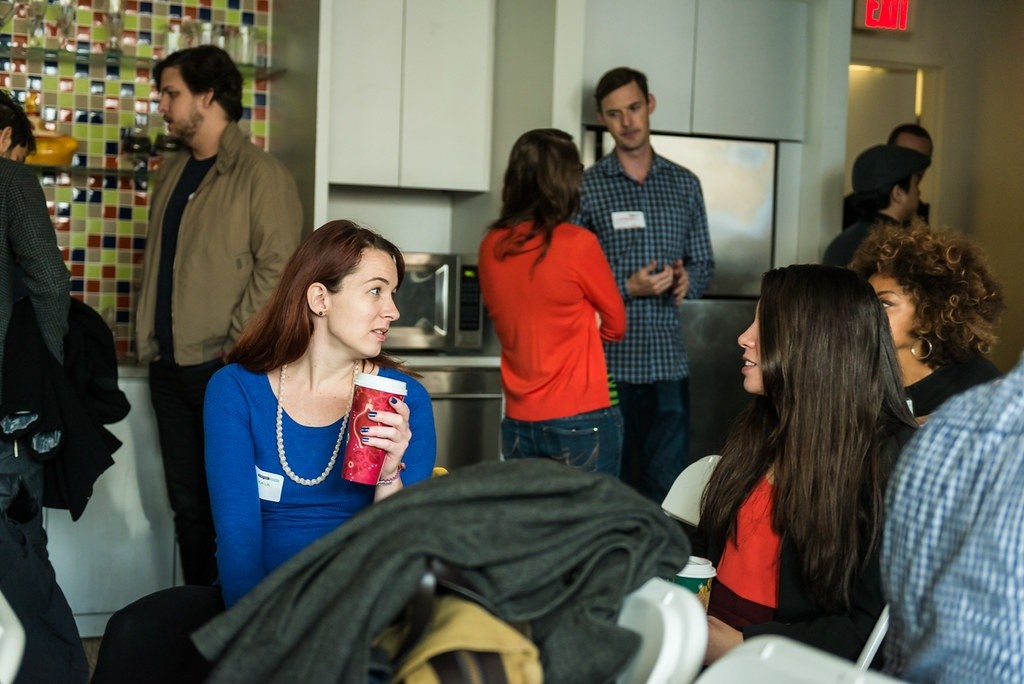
[325,0,495,196]
[38,378,184,640]
[580,0,809,141]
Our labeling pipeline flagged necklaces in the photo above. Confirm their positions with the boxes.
[277,358,360,486]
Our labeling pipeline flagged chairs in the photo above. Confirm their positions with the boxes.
[604,582,888,684]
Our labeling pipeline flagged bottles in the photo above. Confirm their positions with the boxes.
[119,127,181,171]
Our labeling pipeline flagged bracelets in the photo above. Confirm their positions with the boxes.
[378,463,406,484]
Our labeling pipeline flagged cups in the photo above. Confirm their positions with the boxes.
[341,371,411,485]
[665,555,717,616]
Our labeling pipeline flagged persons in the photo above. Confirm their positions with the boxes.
[479,128,627,483]
[573,67,717,501]
[203,220,437,610]
[0,91,132,684]
[135,45,305,586]
[691,122,1024,683]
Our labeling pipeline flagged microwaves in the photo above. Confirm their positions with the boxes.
[376,250,485,351]
